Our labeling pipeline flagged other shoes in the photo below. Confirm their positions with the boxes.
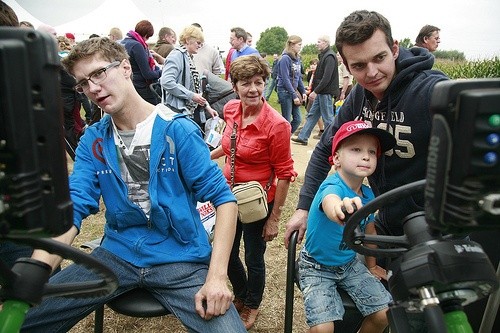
[291,138,308,145]
[314,130,325,139]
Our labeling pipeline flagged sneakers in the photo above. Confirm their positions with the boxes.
[239,304,259,330]
[232,297,246,312]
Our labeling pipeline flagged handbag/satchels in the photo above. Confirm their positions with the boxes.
[165,103,185,114]
[231,180,269,223]
[293,89,303,106]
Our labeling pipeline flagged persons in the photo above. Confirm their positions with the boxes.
[291,38,339,145]
[0,0,176,161]
[192,23,261,80]
[209,55,298,329]
[284,9,452,333]
[19,37,247,333]
[302,52,354,139]
[265,54,279,100]
[413,25,441,53]
[299,121,393,333]
[161,25,219,127]
[277,35,307,141]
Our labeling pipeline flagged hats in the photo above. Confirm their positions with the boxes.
[66,33,75,39]
[328,121,397,163]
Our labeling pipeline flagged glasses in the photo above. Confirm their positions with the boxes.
[72,60,120,94]
[196,41,204,47]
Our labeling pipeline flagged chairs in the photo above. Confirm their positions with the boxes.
[95,294,170,333]
[285,219,391,333]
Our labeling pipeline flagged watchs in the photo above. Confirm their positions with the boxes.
[272,211,280,221]
